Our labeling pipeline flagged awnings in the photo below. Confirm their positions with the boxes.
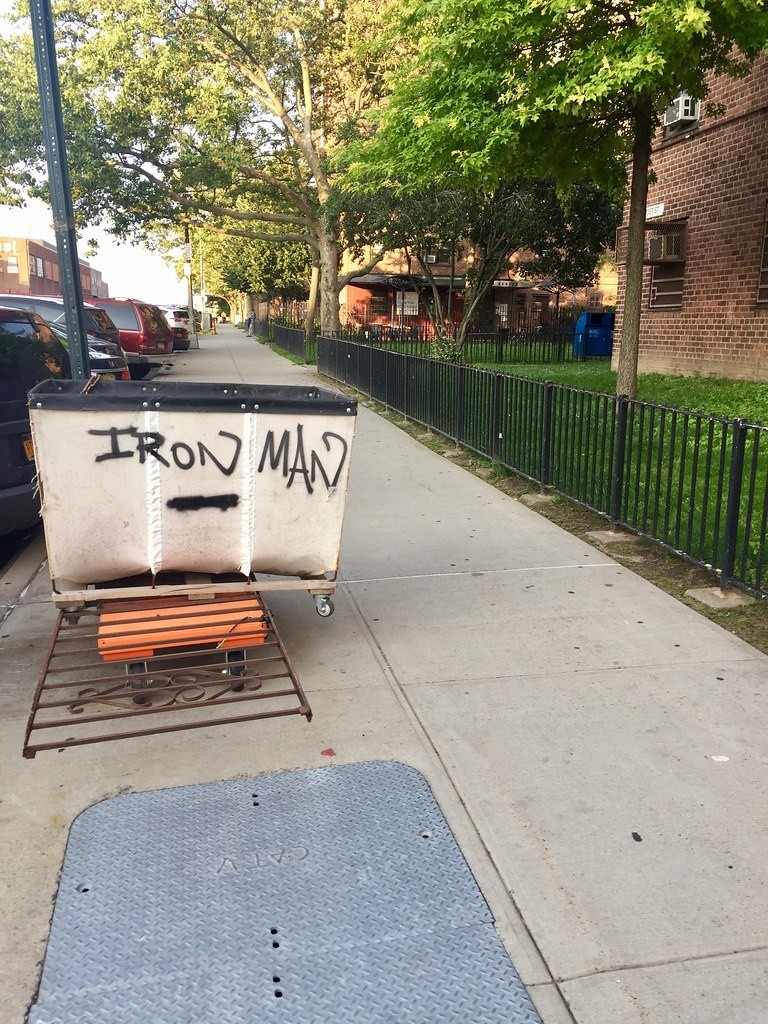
[343,274,534,288]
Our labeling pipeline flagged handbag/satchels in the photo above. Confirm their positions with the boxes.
[248,319,251,324]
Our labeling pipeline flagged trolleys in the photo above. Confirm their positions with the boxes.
[92,571,269,706]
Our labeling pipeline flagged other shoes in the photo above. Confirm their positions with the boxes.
[246,335,252,337]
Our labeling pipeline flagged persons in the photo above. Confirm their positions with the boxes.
[246,309,256,337]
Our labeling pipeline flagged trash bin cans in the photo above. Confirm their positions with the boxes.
[573,311,615,359]
[28,376,359,584]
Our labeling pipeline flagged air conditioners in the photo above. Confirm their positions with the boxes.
[663,97,696,127]
[424,256,436,264]
[648,235,680,261]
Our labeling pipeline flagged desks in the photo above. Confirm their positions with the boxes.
[384,323,411,342]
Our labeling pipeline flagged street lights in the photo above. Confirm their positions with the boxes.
[181,192,200,349]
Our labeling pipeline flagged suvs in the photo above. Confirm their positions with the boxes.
[0,293,132,380]
[154,303,201,352]
[26,294,175,379]
[0,305,71,542]
